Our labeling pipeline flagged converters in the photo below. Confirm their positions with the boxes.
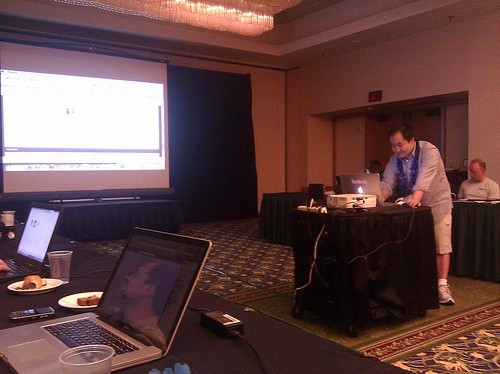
[201,310,245,339]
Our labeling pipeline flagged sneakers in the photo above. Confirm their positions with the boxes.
[439,285,455,305]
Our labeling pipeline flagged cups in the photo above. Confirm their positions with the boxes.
[58,344,115,374]
[48,251,73,284]
[2,210,16,226]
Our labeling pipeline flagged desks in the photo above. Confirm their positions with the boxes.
[294,203,439,335]
[0,220,415,374]
[450,198,500,283]
[259,191,334,245]
[39,198,179,238]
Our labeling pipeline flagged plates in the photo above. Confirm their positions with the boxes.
[58,291,104,310]
[7,279,63,295]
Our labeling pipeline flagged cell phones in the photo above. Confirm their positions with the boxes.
[9,307,55,321]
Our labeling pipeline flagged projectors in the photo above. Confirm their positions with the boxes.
[327,194,377,209]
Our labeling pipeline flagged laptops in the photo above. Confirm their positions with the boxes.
[0,202,64,283]
[0,227,212,374]
[340,173,398,207]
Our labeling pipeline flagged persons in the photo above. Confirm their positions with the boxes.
[457,159,499,200]
[381,124,455,305]
[123,257,177,328]
[368,160,382,181]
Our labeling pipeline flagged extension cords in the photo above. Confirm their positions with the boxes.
[297,205,326,213]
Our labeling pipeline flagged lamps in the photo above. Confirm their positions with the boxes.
[55,0,275,38]
